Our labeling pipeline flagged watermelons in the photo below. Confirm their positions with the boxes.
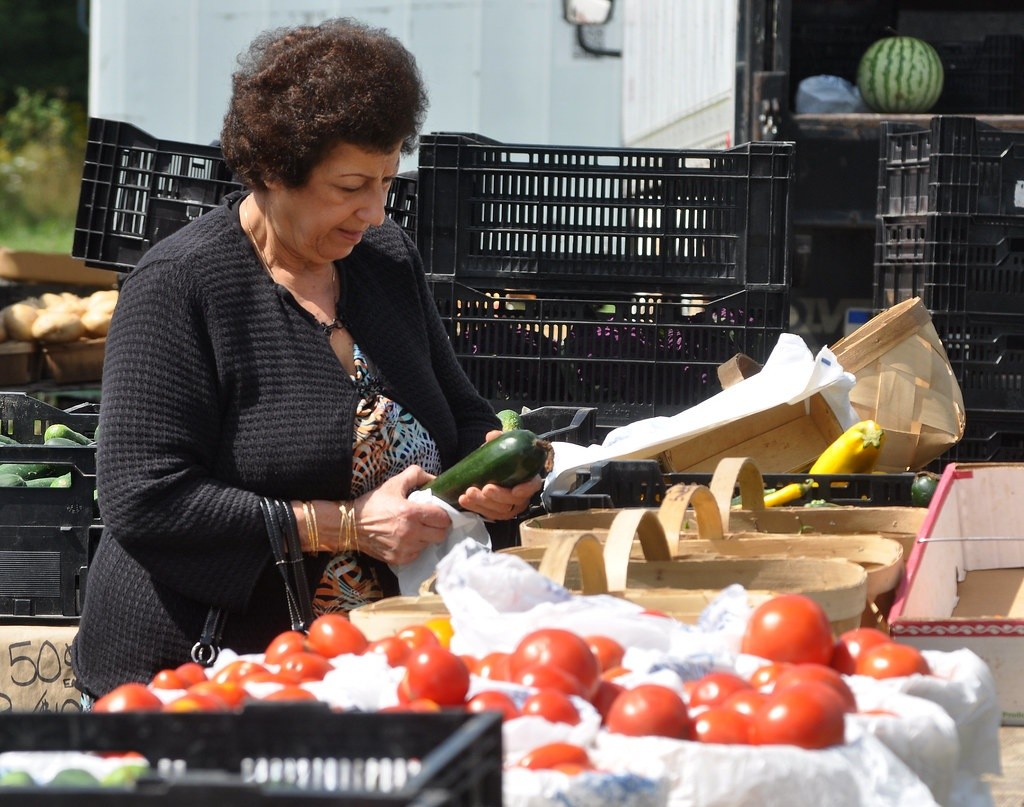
[856,35,944,113]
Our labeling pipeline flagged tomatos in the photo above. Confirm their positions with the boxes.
[95,594,932,776]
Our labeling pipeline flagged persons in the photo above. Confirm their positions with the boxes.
[68,16,543,710]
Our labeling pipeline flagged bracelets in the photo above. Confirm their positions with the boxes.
[300,499,361,558]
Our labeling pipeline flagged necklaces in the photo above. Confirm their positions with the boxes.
[245,193,336,339]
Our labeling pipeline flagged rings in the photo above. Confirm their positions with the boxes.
[508,504,514,512]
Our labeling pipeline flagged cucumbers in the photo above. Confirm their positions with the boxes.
[910,474,937,508]
[408,430,544,509]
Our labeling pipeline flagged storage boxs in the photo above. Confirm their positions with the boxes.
[72,118,417,274]
[551,460,915,510]
[415,132,800,553]
[0,252,122,386]
[0,390,105,626]
[787,35,1024,474]
[886,460,1023,727]
[0,710,506,807]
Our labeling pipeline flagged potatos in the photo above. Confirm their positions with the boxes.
[0,289,120,342]
